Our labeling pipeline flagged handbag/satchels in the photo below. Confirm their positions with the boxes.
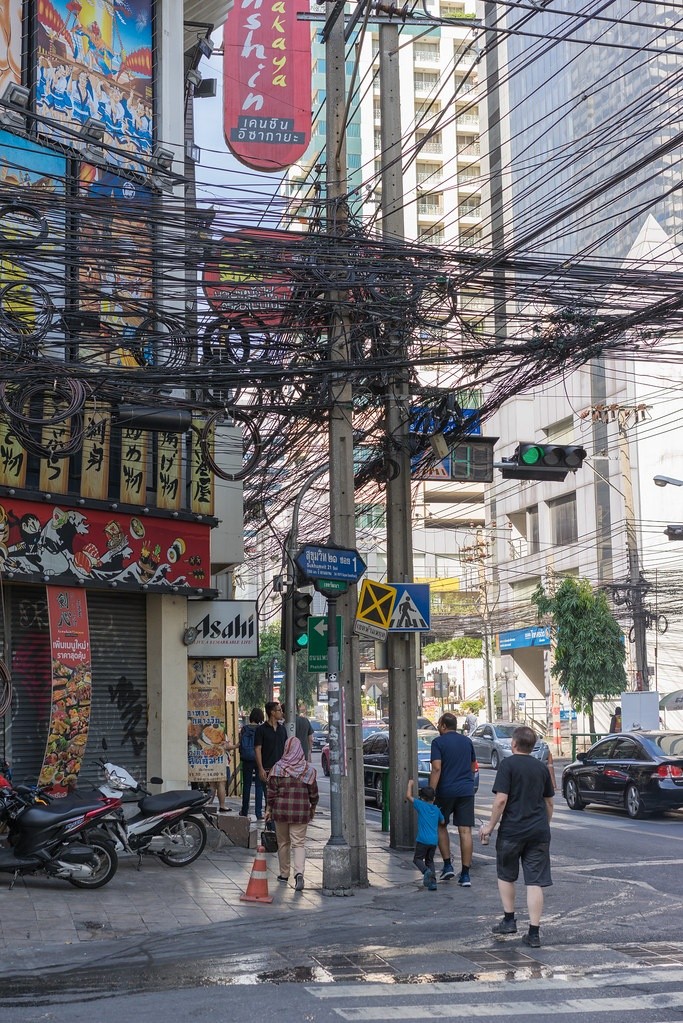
[261,817,278,853]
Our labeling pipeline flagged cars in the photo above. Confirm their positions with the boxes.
[562,731,683,819]
[309,716,479,810]
[468,722,551,769]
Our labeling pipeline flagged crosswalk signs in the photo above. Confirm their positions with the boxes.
[386,583,431,631]
[411,446,450,479]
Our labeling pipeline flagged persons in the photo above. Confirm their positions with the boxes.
[610,707,621,733]
[429,713,477,887]
[265,736,320,891]
[208,734,240,812]
[254,702,288,831]
[479,726,555,947]
[281,703,314,761]
[406,780,445,891]
[466,708,477,733]
[239,708,264,820]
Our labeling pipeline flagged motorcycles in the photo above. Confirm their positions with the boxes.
[91,738,213,872]
[0,760,125,889]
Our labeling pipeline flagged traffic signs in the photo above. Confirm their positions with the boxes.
[307,615,343,673]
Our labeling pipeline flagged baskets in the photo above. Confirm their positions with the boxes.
[67,779,97,797]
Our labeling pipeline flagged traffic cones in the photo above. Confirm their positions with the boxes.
[239,846,274,904]
[547,753,557,790]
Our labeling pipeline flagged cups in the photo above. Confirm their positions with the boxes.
[480,823,489,845]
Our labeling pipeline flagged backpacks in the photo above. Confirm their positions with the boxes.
[241,725,257,761]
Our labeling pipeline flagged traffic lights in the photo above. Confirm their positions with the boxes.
[292,592,314,653]
[516,442,587,468]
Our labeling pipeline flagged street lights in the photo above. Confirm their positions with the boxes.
[495,667,519,722]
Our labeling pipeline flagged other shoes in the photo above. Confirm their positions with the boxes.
[277,876,288,882]
[429,882,437,890]
[295,873,304,890]
[424,868,431,887]
[219,808,232,812]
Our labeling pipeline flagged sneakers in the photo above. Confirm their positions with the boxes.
[456,875,472,886]
[522,934,541,947]
[439,864,455,880]
[492,917,518,933]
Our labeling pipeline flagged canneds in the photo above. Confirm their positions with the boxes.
[480,824,489,844]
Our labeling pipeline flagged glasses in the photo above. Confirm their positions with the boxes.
[275,709,282,713]
